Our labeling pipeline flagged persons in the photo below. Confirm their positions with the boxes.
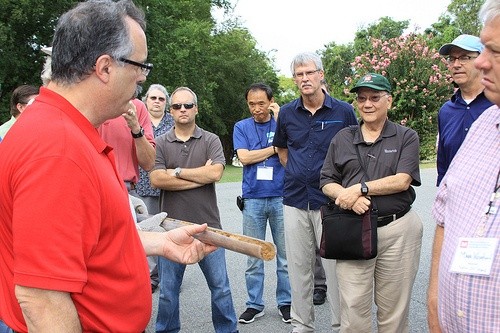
[232,83,292,323]
[95,98,157,202]
[124,84,176,293]
[319,72,422,333]
[0,84,39,143]
[435,34,496,187]
[149,88,241,333]
[311,80,331,305]
[425,0,500,333]
[271,51,358,333]
[0,0,217,333]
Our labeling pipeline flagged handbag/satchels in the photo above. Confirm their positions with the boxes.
[317,203,379,260]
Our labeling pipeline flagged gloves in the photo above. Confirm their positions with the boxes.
[128,194,147,215]
[132,212,168,234]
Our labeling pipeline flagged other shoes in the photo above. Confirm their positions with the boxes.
[278,306,291,323]
[313,288,327,305]
[238,308,265,323]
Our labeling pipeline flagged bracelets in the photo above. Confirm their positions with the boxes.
[273,146,276,154]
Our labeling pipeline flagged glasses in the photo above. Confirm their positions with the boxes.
[150,97,165,101]
[445,55,478,66]
[120,57,153,76]
[172,103,195,109]
[355,94,386,103]
[294,69,318,77]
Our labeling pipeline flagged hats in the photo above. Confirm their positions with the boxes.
[439,34,484,54]
[349,73,391,93]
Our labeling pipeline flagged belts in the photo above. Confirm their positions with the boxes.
[377,206,411,228]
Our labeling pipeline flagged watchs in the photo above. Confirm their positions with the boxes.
[175,167,182,176]
[360,182,369,196]
[131,126,145,138]
